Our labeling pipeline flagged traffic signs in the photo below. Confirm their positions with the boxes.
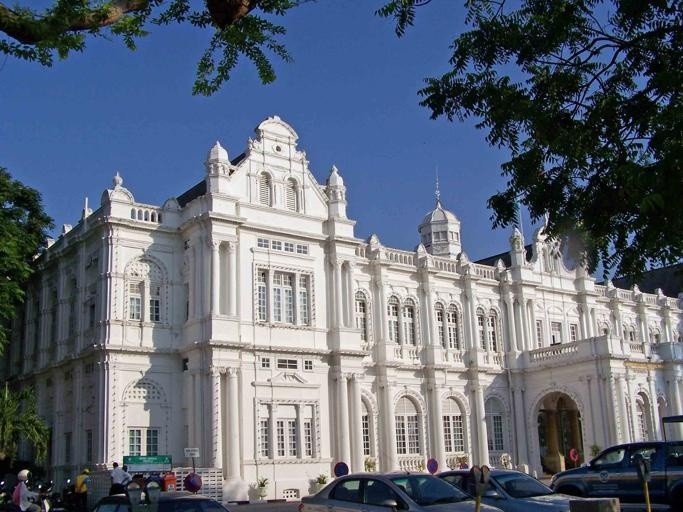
[183,447,200,458]
[122,455,172,473]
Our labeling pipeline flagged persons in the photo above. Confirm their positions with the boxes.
[74,466,90,506]
[108,461,128,496]
[119,466,131,487]
[10,467,40,511]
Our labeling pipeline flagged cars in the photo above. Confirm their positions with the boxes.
[420,466,611,512]
[88,489,231,511]
[294,468,503,510]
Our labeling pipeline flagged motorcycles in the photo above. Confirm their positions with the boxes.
[1,479,77,511]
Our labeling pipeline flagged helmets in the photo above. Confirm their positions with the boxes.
[81,469,89,474]
[18,469,33,484]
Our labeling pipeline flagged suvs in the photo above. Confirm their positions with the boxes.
[547,437,683,506]
